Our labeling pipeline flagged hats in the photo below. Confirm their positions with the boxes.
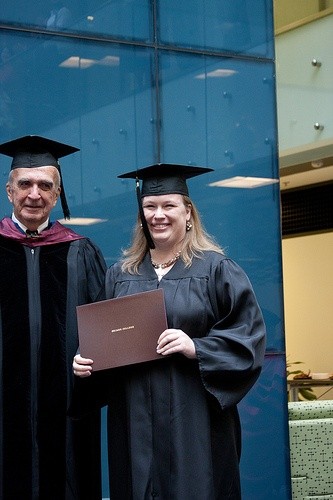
[117,163,214,250]
[0,135,80,219]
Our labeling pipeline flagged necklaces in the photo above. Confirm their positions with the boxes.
[150,249,181,269]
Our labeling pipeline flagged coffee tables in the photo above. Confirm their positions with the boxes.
[286,373,333,401]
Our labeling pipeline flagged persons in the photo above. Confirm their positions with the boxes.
[0,153,108,500]
[72,179,267,500]
